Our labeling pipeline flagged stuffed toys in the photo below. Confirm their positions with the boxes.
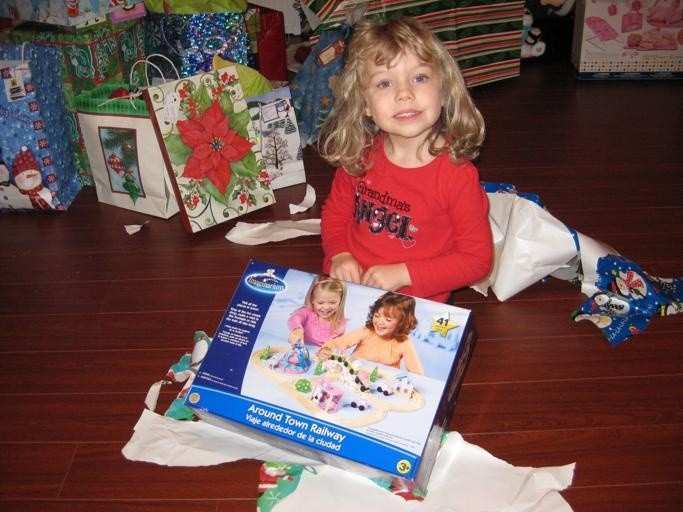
[519,10,545,58]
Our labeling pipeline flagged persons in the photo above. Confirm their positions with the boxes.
[316,16,495,305]
[309,287,429,379]
[286,276,348,356]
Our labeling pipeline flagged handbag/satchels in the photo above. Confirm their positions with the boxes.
[75,53,180,220]
[0,41,85,216]
[141,2,288,87]
[248,98,307,191]
